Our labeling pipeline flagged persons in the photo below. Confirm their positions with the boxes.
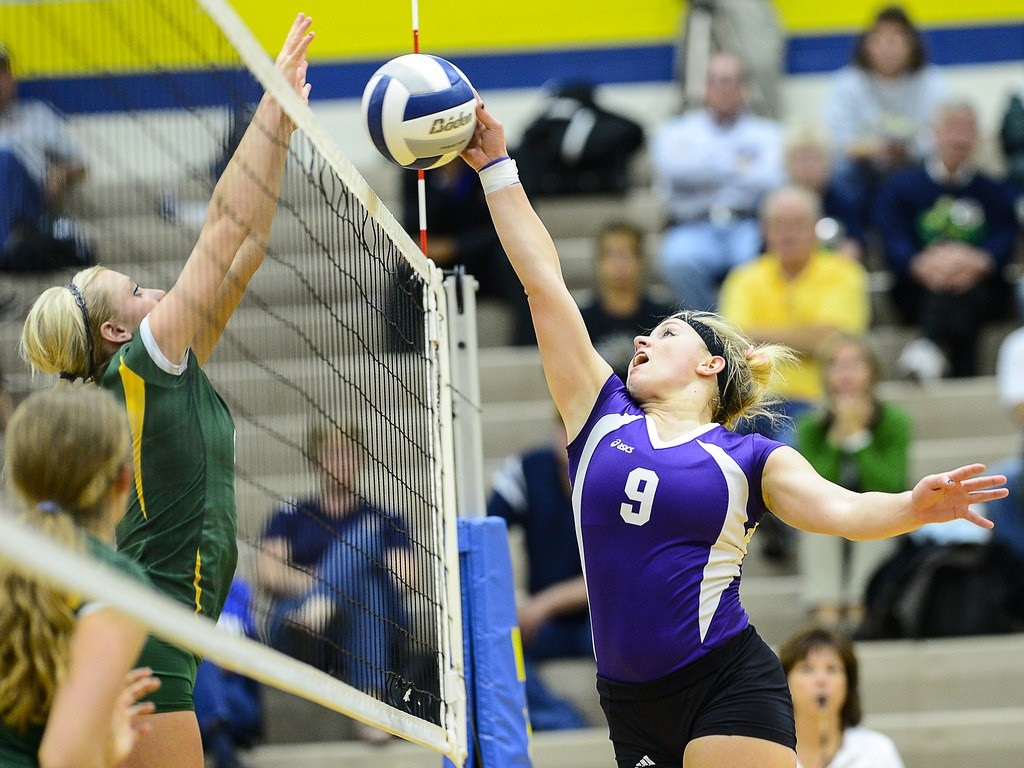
[377,139,537,351]
[253,407,419,752]
[0,374,164,768]
[468,0,1024,768]
[0,8,319,768]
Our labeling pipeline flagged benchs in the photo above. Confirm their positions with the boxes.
[0,56,1024,768]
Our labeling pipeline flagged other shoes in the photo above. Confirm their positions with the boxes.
[896,335,947,380]
[350,722,394,743]
[284,612,321,670]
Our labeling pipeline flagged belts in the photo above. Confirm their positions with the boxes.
[675,204,758,227]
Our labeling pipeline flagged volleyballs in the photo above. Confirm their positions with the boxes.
[358,51,481,173]
